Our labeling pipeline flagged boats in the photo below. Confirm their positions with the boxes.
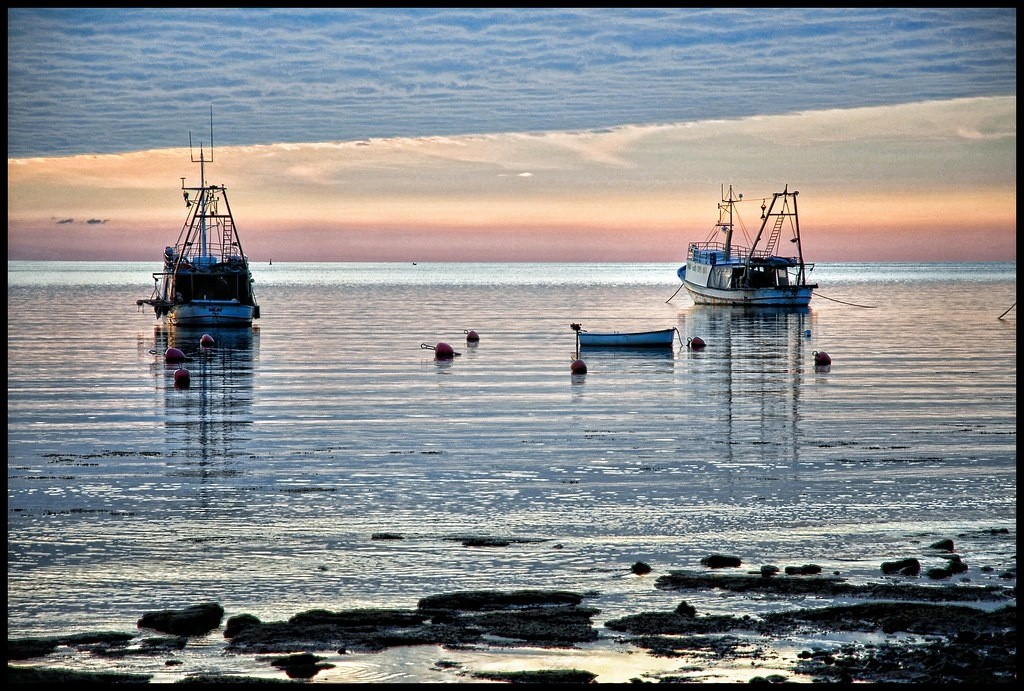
[676,182,819,306]
[570,320,677,347]
[132,103,262,326]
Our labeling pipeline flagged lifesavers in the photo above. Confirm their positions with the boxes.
[175,255,189,265]
